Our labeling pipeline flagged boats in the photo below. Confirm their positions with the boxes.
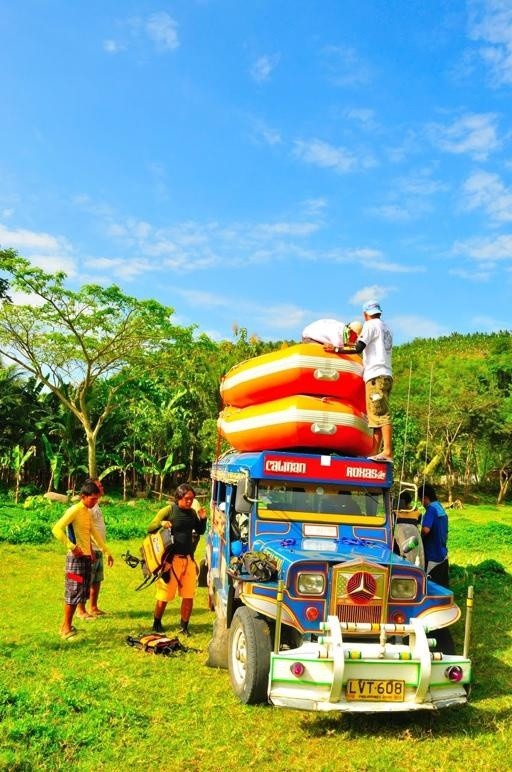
[213,339,382,453]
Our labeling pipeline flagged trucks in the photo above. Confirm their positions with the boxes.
[206,452,473,718]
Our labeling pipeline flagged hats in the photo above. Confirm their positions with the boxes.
[362,301,382,316]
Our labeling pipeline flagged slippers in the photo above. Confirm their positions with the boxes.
[367,454,393,463]
[78,612,107,620]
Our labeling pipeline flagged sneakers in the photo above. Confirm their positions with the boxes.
[178,626,191,637]
[153,622,165,633]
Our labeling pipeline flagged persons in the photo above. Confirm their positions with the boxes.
[302,301,395,464]
[393,485,455,655]
[52,478,113,642]
[147,483,208,637]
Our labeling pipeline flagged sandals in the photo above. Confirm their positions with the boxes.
[60,623,85,643]
